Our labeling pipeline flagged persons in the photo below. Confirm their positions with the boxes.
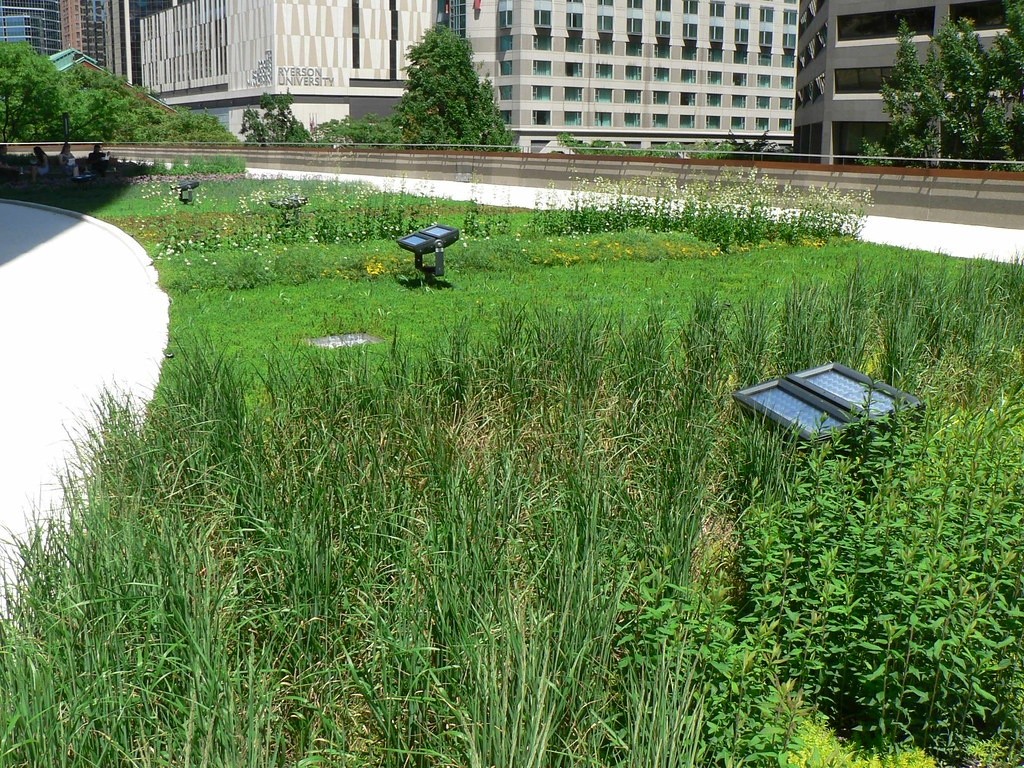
[29,147,50,186]
[88,145,109,177]
[0,144,20,188]
[57,143,80,177]
